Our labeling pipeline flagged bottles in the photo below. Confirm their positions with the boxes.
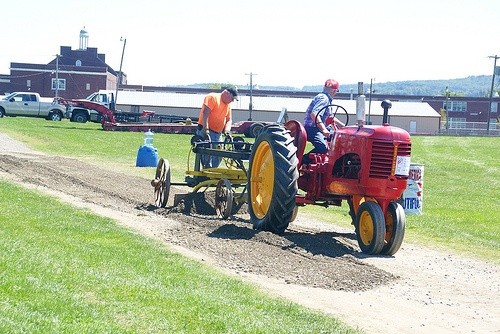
[144,128,155,145]
[136,144,159,167]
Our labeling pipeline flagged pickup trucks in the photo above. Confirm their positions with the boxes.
[0,92,66,122]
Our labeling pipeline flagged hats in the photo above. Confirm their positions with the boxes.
[325,79,339,92]
[227,87,239,101]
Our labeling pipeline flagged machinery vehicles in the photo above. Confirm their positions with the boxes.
[154,98,414,257]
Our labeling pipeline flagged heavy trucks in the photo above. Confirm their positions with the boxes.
[70,90,213,128]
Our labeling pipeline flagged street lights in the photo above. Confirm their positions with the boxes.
[246,72,258,121]
[113,36,127,109]
[486,55,500,134]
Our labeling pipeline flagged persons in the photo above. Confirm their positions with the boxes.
[302,80,347,166]
[196,86,240,168]
